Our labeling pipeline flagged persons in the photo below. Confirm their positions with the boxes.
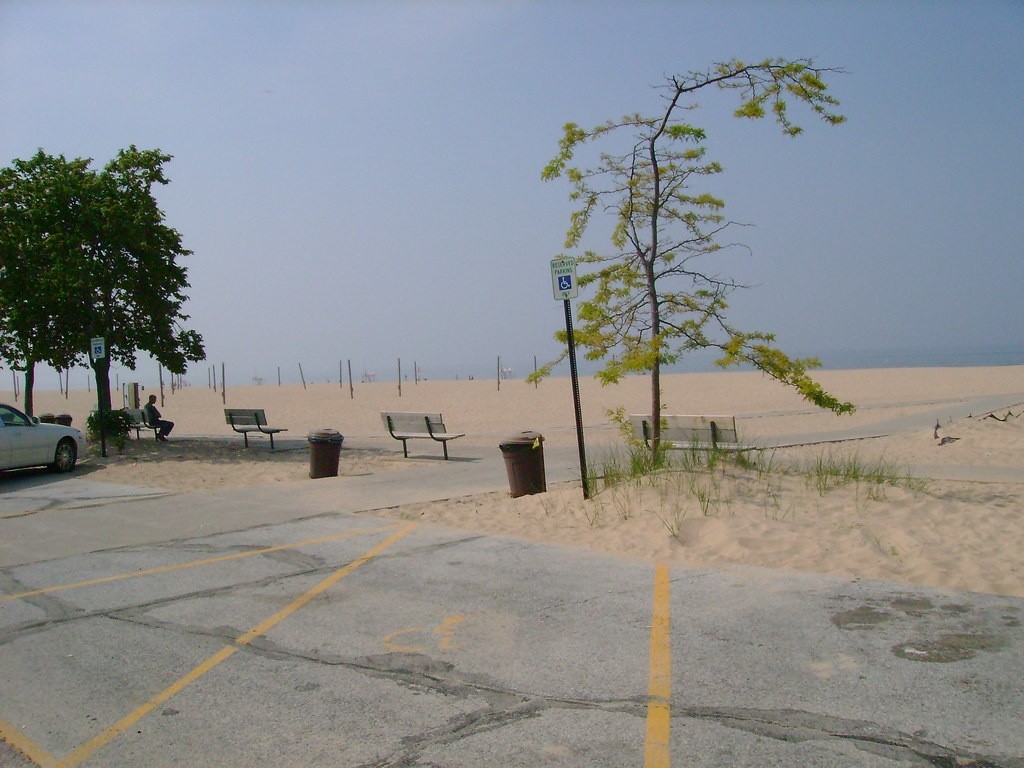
[144,394,174,441]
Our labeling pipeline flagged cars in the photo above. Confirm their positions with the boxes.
[0,403,87,474]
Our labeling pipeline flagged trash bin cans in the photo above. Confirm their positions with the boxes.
[306,428,344,480]
[39,413,55,424]
[499,429,547,499]
[54,414,73,427]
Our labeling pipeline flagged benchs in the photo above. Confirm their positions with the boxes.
[223,408,288,450]
[379,411,465,461]
[628,413,756,466]
[117,409,162,443]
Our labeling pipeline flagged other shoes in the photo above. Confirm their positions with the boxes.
[156,433,168,441]
